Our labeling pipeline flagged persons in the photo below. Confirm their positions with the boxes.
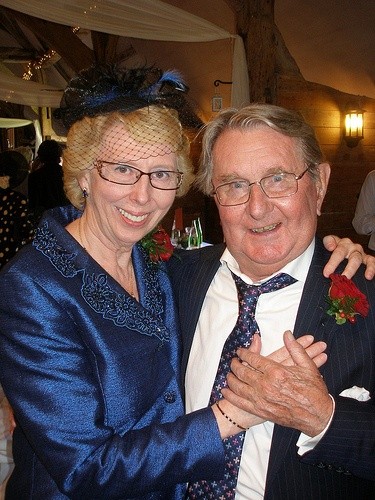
[163,104,375,500]
[0,138,64,265]
[0,102,375,500]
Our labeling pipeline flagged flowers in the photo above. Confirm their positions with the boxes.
[140,224,182,275]
[323,274,370,325]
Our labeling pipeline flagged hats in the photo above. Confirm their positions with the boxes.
[51,63,188,136]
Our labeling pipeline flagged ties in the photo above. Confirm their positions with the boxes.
[185,272,299,500]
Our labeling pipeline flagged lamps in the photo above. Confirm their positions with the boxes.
[342,96,366,148]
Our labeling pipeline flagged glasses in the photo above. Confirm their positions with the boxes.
[212,164,312,206]
[96,159,183,190]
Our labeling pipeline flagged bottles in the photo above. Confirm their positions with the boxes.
[172,217,203,249]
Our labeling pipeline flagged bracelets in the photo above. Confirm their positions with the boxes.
[214,401,250,432]
